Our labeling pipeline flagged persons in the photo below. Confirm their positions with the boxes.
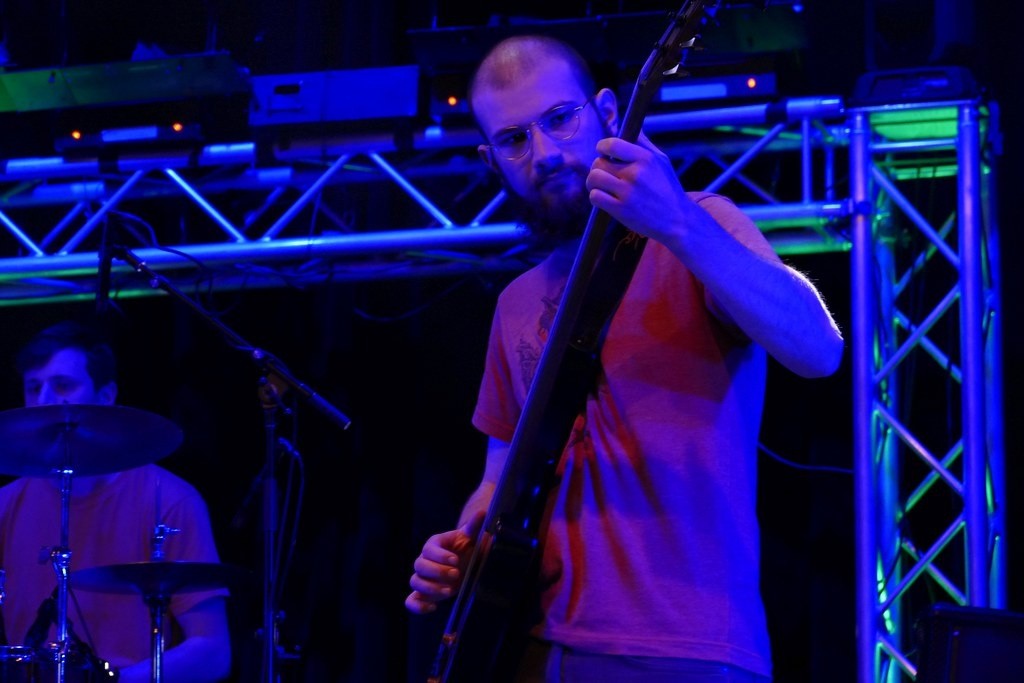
[0,322,232,683]
[405,35,845,683]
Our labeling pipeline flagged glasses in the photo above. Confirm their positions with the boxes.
[481,89,599,161]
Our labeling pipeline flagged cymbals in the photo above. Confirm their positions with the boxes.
[0,402,185,480]
[68,560,241,595]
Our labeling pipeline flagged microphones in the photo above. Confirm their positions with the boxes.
[23,586,59,648]
[96,211,115,311]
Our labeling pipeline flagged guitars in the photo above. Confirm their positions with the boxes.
[424,0,735,683]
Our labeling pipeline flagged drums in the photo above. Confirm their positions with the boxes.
[0,644,121,682]
